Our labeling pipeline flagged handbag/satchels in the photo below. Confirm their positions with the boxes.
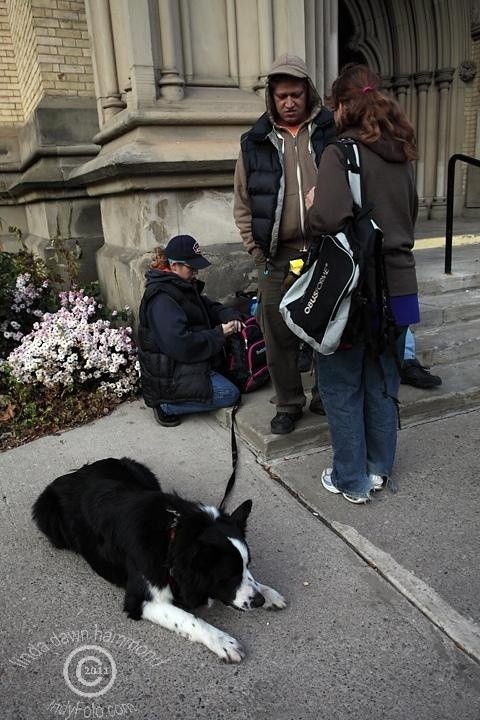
[279,203,384,355]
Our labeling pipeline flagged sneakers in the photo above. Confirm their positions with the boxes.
[152,404,180,426]
[309,400,327,415]
[321,468,385,504]
[271,411,303,434]
[399,358,442,388]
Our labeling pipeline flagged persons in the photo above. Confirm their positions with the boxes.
[397,324,444,389]
[303,63,417,507]
[230,52,340,434]
[135,233,249,428]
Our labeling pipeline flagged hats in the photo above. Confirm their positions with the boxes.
[268,68,306,78]
[165,235,211,270]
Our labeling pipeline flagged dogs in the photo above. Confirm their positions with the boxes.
[31,456,288,666]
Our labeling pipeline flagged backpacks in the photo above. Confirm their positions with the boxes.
[216,310,270,394]
